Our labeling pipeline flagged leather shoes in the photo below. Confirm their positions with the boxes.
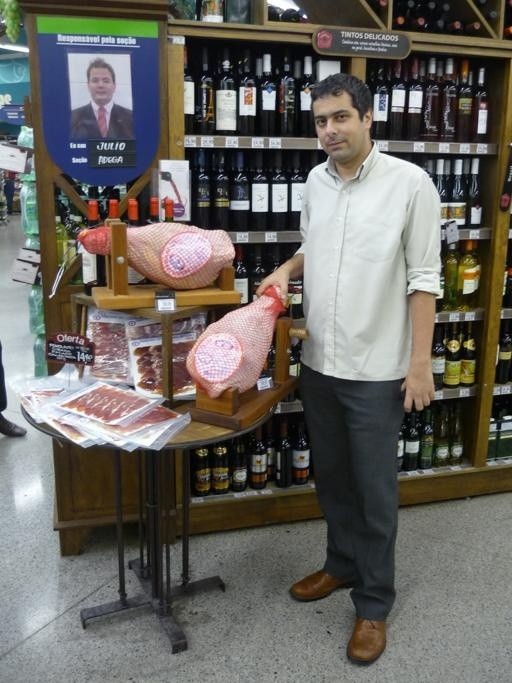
[289,569,356,602]
[348,616,386,664]
[0,413,27,438]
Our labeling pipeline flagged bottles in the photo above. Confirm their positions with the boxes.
[266,321,480,392]
[80,195,175,296]
[184,151,487,230]
[12,125,48,387]
[191,402,470,497]
[234,240,483,315]
[496,254,511,386]
[372,0,512,40]
[168,0,252,26]
[182,32,496,144]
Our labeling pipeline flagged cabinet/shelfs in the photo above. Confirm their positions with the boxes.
[19,1,512,557]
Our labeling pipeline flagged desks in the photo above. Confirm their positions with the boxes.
[21,379,283,654]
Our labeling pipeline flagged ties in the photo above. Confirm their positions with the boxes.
[95,105,110,138]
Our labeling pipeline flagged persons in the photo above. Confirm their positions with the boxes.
[0,340,26,436]
[71,56,134,140]
[258,73,442,666]
[4,179,15,214]
